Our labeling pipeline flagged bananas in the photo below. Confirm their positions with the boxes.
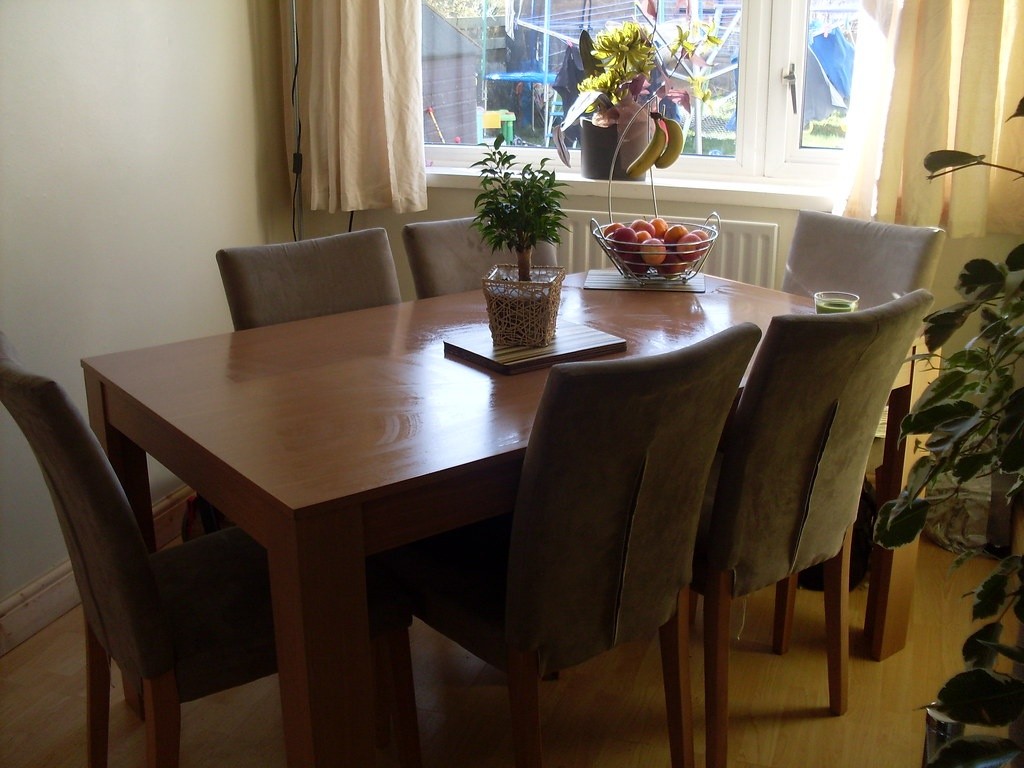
[627,113,685,177]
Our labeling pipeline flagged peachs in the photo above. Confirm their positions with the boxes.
[602,217,711,278]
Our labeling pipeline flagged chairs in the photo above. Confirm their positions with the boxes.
[387,324,763,768]
[690,292,934,768]
[404,205,560,304]
[781,209,948,312]
[216,224,403,335]
[1,332,413,768]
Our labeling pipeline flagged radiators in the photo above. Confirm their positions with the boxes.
[558,207,779,289]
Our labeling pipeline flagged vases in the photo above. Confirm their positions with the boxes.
[577,116,646,182]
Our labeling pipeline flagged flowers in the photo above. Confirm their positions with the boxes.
[553,1,722,166]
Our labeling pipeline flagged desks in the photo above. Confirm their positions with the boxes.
[79,267,870,768]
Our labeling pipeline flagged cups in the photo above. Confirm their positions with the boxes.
[813,291,860,314]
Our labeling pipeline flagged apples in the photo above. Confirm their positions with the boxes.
[676,233,704,261]
[629,219,655,236]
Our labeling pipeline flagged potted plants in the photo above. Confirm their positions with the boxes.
[468,134,573,347]
[872,99,1024,768]
